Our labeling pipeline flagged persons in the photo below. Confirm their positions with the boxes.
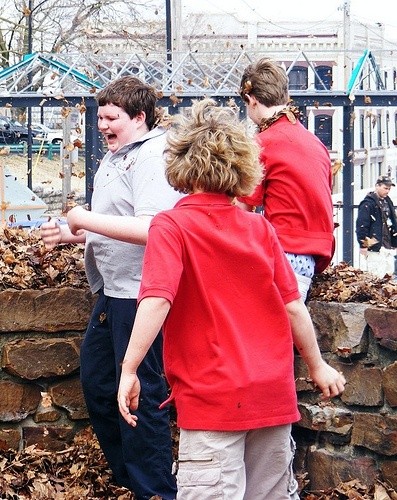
[40,76,188,500]
[238,55,334,305]
[354,177,397,278]
[117,97,348,500]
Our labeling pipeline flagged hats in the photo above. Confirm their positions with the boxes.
[376,176,395,186]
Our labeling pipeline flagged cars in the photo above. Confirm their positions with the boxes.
[0,117,69,145]
